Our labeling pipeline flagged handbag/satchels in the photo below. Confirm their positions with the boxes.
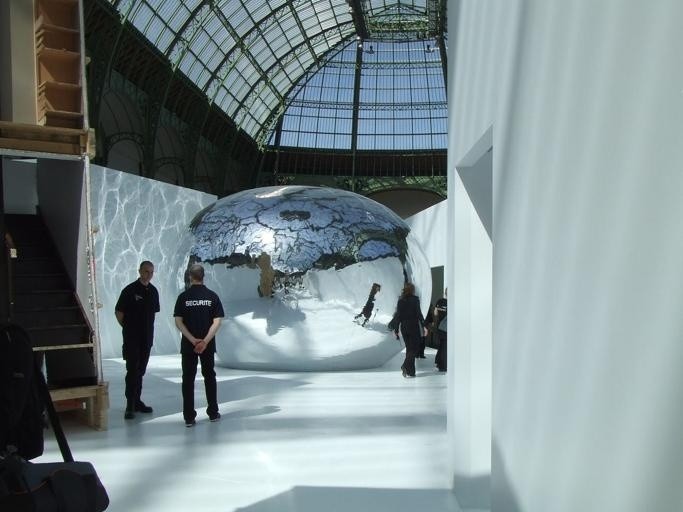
[0,461,109,512]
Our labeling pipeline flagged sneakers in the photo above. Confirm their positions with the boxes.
[186,419,194,426]
[210,414,220,422]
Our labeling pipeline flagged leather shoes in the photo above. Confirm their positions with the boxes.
[137,406,151,412]
[125,408,135,418]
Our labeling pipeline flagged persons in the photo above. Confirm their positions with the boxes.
[115,261,160,418]
[394,283,447,378]
[173,265,224,426]
